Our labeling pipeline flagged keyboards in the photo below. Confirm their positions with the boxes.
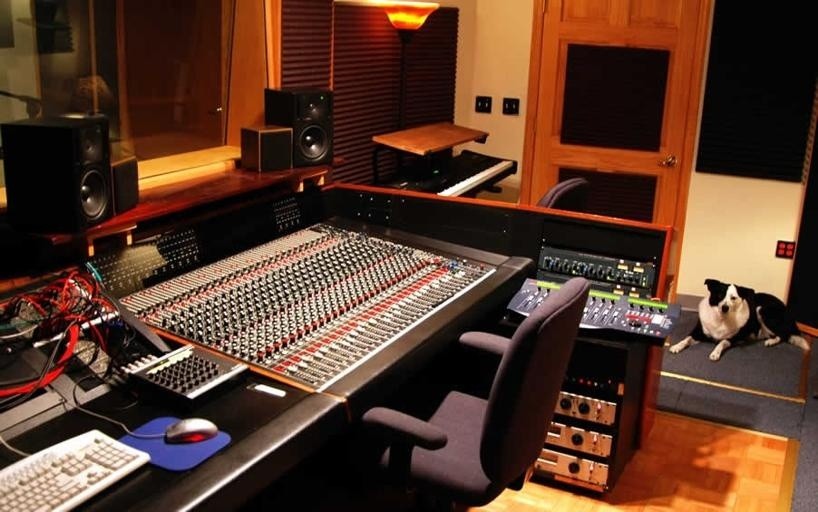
[0,428,150,512]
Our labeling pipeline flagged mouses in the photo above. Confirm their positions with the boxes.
[166,416,218,445]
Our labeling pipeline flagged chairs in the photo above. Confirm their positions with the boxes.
[361,275,589,512]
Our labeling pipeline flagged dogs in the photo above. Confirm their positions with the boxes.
[666,277,812,362]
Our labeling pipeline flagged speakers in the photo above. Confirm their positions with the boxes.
[242,124,294,172]
[1,114,112,230]
[266,83,333,170]
[112,157,139,213]
